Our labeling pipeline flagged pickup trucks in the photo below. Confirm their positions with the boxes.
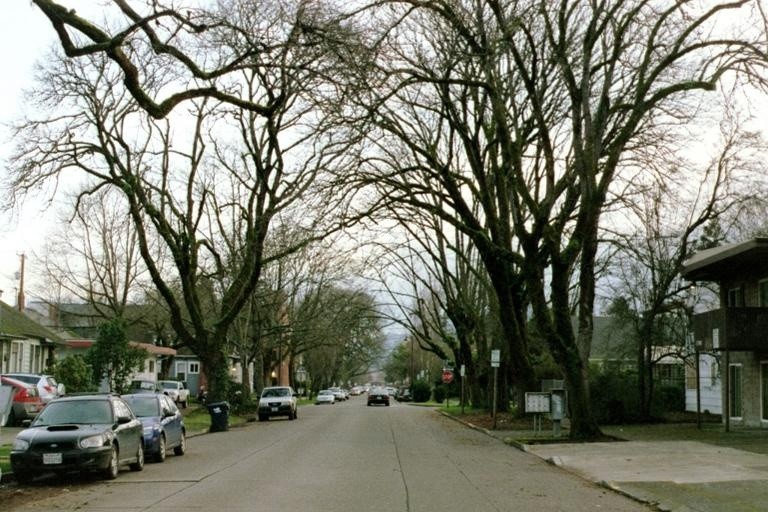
[158,379,192,409]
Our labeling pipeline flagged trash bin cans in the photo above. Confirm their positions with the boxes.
[207,401,230,432]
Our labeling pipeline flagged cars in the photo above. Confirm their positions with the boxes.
[127,378,164,396]
[1,375,43,428]
[314,383,413,407]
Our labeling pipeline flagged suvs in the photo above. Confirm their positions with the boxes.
[10,389,145,483]
[118,392,190,465]
[254,384,299,422]
[2,373,67,407]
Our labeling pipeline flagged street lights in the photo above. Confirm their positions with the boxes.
[277,326,293,386]
[404,333,414,401]
